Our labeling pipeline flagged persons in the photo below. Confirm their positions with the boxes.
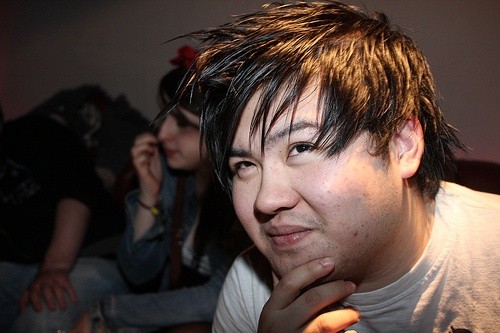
[77,48,240,333]
[188,1,500,333]
[0,116,126,333]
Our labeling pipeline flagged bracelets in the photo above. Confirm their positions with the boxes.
[134,196,160,215]
